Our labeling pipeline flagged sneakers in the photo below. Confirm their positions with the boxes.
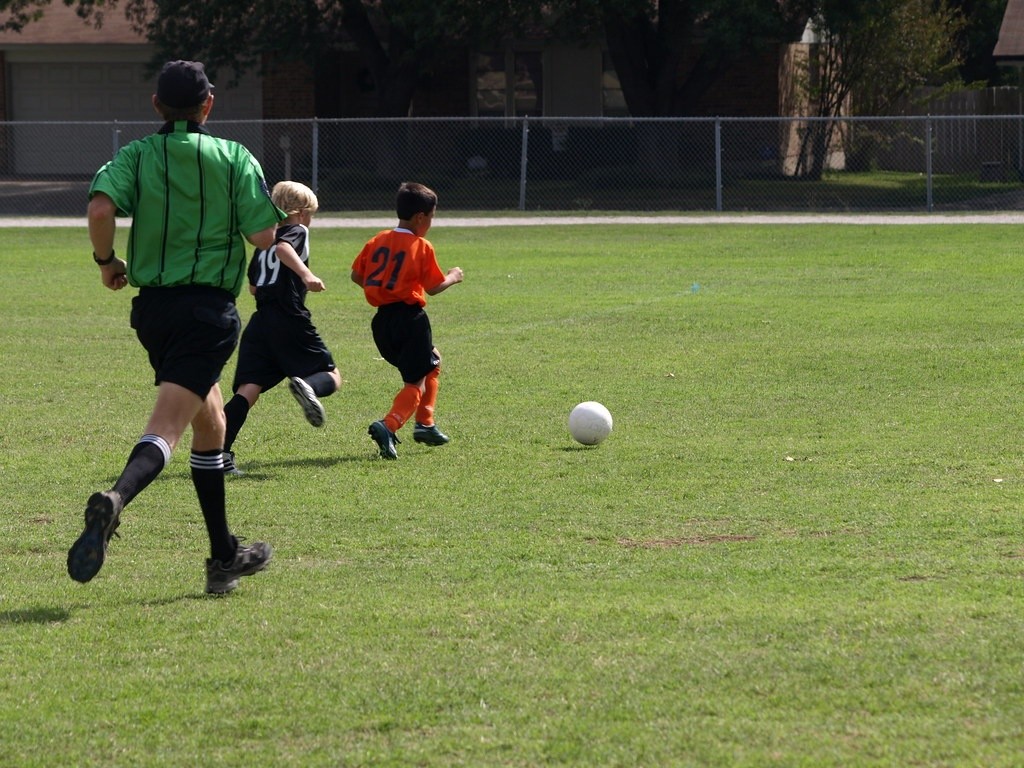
[221,452,245,477]
[368,420,401,459]
[206,536,272,594]
[288,377,325,427]
[67,491,123,583]
[414,423,449,445]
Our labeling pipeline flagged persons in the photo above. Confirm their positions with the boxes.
[350,183,464,461]
[222,181,340,475]
[65,60,289,594]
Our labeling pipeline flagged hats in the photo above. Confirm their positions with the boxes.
[157,60,215,109]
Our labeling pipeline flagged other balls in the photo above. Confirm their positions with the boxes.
[567,400,614,445]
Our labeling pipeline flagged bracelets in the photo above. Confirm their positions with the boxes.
[93,249,115,266]
